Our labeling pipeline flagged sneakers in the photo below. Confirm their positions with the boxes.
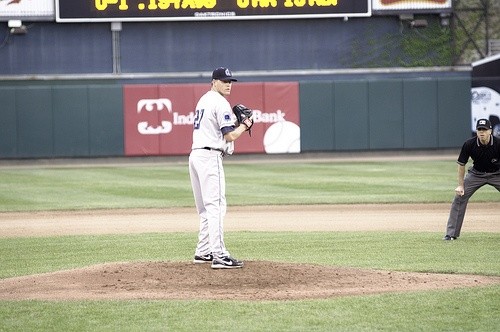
[194,252,213,263]
[211,256,244,268]
[442,235,456,240]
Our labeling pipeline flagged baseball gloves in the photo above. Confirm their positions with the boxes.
[231,103,254,131]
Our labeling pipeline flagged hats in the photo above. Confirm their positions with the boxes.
[476,119,492,129]
[212,67,237,82]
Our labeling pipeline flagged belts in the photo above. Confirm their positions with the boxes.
[202,147,224,157]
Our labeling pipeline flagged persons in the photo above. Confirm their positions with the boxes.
[189,67,253,269]
[442,119,500,240]
